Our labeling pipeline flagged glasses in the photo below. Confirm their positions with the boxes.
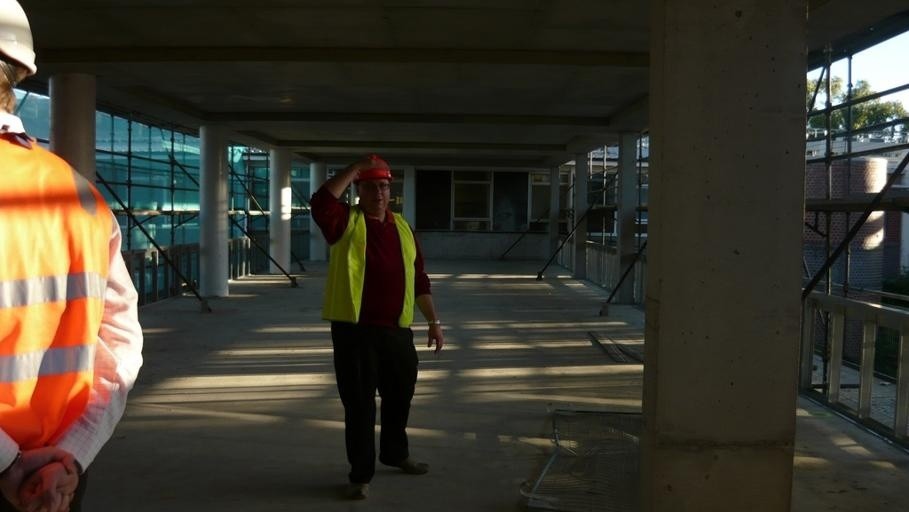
[359,183,390,191]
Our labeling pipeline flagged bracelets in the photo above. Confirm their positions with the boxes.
[428,319,441,325]
[1,451,22,479]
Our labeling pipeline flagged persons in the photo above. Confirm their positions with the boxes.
[308,155,445,500]
[0,1,147,512]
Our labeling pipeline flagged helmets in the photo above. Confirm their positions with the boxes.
[354,156,395,181]
[2,1,39,75]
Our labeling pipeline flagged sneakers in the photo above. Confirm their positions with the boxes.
[380,453,430,475]
[346,483,368,500]
